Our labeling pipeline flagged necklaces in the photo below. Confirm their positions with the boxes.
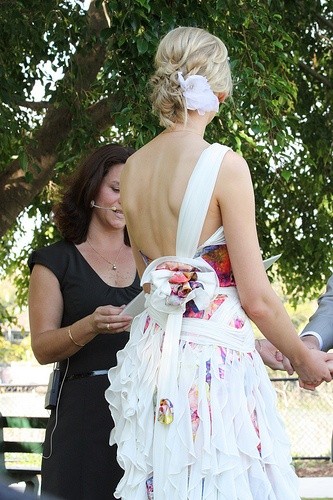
[85,239,124,270]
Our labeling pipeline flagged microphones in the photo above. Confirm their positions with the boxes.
[90,200,116,210]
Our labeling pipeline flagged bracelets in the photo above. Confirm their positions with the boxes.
[69,325,85,347]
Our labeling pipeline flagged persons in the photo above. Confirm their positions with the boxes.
[27,143,137,500]
[275,274,333,392]
[120,25,333,500]
